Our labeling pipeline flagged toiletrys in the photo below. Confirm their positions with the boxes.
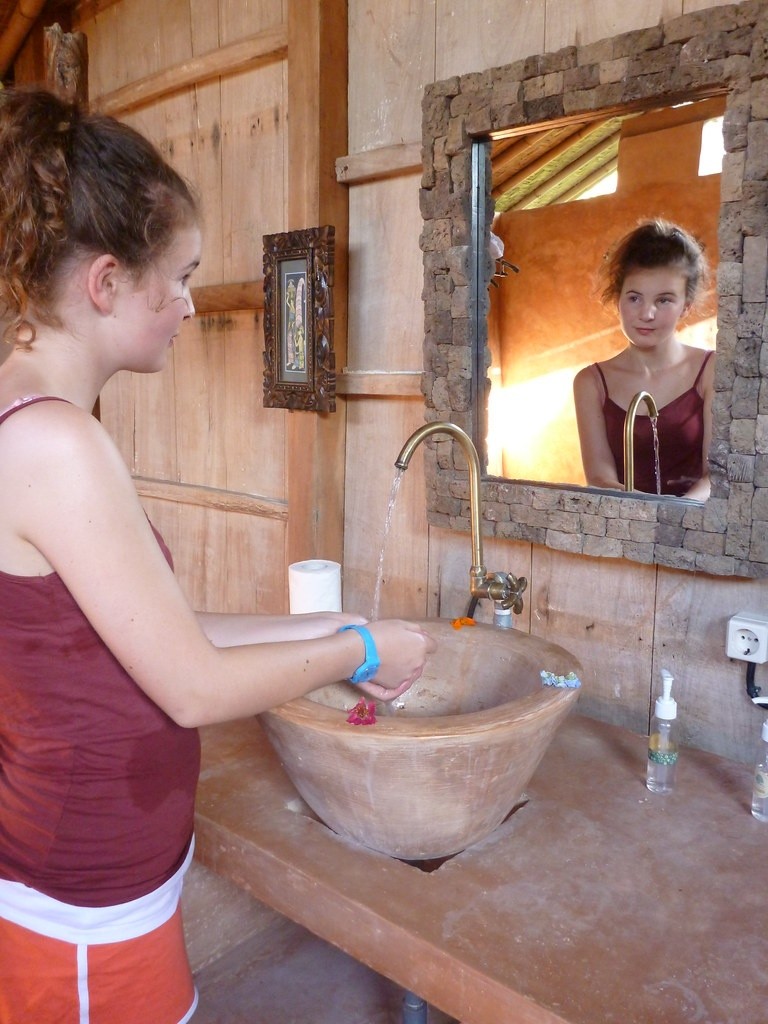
[751,695,768,824]
[646,669,679,794]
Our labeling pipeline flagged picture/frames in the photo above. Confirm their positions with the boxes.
[261,223,338,411]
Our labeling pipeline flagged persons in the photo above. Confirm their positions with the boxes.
[573,223,716,501]
[0,88,437,1024]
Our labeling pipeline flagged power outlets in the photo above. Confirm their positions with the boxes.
[725,610,768,664]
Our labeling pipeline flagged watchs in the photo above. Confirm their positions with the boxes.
[339,624,380,684]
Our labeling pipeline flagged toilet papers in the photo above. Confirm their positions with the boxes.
[288,559,342,619]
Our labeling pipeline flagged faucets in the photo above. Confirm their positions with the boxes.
[624,391,658,493]
[396,422,527,617]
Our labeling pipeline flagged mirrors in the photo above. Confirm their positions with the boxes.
[477,92,728,501]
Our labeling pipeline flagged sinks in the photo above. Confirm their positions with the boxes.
[256,617,583,859]
